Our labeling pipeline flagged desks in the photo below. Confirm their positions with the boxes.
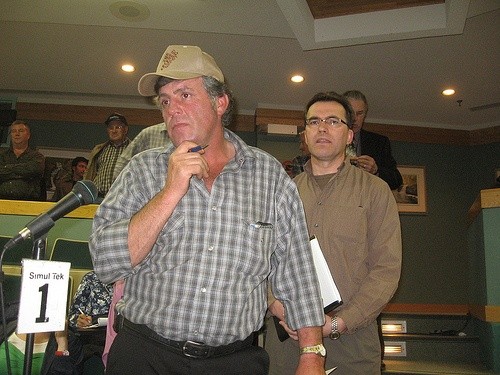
[0,200,102,240]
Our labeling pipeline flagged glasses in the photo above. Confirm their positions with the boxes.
[304,117,351,129]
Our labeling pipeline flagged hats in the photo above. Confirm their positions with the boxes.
[138,45,225,96]
[105,112,128,126]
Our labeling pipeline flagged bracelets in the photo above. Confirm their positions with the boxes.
[373,169,378,175]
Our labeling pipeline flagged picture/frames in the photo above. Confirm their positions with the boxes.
[391,165,430,216]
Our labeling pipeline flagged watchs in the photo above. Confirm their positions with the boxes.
[330,315,341,340]
[299,344,327,357]
[55,350,70,356]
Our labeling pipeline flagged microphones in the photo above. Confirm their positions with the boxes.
[3,180,98,253]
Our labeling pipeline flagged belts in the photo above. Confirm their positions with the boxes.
[117,311,254,359]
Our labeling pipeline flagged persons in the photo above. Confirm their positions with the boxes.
[88,45,327,375]
[85,112,131,199]
[265,92,402,375]
[0,121,47,201]
[281,128,311,179]
[114,85,236,184]
[51,157,89,202]
[68,265,118,328]
[343,90,403,190]
[0,274,105,375]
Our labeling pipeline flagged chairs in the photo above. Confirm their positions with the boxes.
[49,238,94,269]
[0,236,34,267]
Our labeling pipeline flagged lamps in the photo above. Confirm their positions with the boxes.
[382,320,408,333]
[384,341,407,357]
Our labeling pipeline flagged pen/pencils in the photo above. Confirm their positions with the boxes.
[186,143,209,153]
[78,307,90,323]
